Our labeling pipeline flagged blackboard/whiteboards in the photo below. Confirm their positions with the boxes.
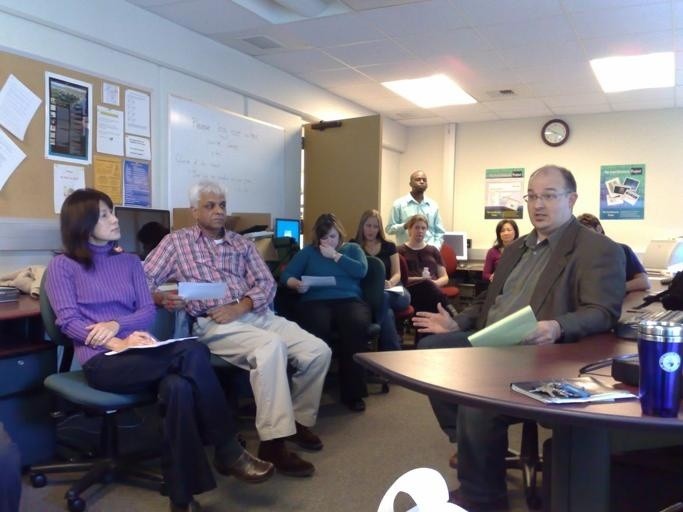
[166,93,288,238]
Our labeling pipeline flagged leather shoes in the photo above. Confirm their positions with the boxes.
[171,500,203,512]
[340,395,364,411]
[216,450,274,483]
[448,489,508,512]
[448,453,459,469]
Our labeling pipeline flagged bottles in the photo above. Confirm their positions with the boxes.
[421,267,430,279]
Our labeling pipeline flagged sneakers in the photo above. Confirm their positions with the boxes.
[258,440,315,477]
[285,421,323,451]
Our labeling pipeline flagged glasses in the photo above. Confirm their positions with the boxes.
[523,191,570,203]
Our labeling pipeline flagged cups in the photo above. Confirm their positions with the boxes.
[635,318,682,419]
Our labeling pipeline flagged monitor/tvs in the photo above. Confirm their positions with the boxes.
[444,231,468,261]
[274,216,301,247]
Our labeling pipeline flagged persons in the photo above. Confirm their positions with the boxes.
[479,219,519,293]
[576,212,653,297]
[394,213,455,350]
[347,207,414,351]
[43,185,277,511]
[382,167,445,253]
[410,163,628,511]
[141,178,333,478]
[277,210,374,413]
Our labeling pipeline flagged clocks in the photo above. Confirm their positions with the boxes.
[540,119,569,147]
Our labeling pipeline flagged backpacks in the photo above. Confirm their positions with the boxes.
[634,271,682,311]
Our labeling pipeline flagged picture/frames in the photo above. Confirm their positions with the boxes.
[44,70,93,165]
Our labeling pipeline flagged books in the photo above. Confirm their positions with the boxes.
[509,375,639,405]
[467,304,539,346]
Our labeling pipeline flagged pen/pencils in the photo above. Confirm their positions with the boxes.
[564,383,590,398]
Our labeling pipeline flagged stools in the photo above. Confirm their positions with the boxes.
[503,417,548,510]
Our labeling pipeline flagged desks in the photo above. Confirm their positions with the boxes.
[352,329,683,512]
[0,292,54,360]
[448,258,488,272]
[618,272,683,333]
